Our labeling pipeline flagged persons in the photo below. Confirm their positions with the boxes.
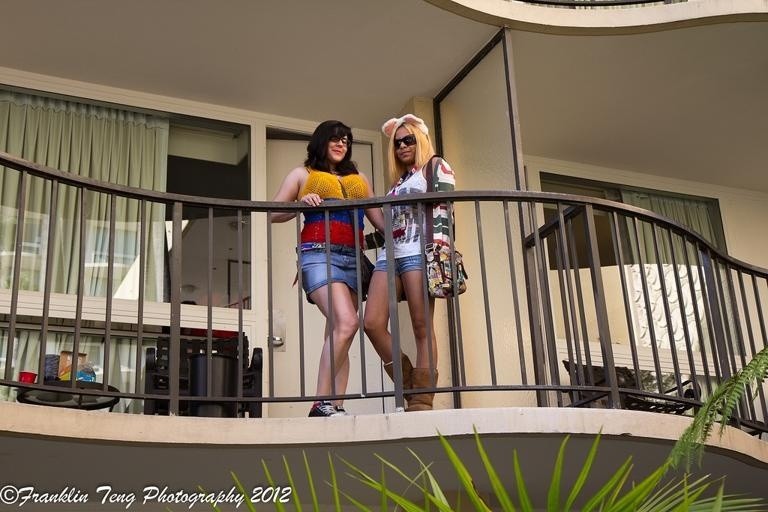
[363,113,457,412]
[271,119,385,418]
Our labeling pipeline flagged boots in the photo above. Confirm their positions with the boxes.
[383,352,438,412]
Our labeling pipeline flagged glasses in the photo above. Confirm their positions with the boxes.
[394,136,417,150]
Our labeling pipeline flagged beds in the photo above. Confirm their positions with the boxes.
[19,369,120,408]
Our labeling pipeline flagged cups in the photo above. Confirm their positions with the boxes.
[18,370,37,387]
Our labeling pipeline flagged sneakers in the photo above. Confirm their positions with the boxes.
[308,399,349,417]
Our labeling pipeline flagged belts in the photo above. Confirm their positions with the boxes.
[300,242,357,257]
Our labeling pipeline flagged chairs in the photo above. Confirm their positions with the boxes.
[143,334,262,419]
[562,362,704,417]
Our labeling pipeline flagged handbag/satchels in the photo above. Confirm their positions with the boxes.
[424,242,466,299]
